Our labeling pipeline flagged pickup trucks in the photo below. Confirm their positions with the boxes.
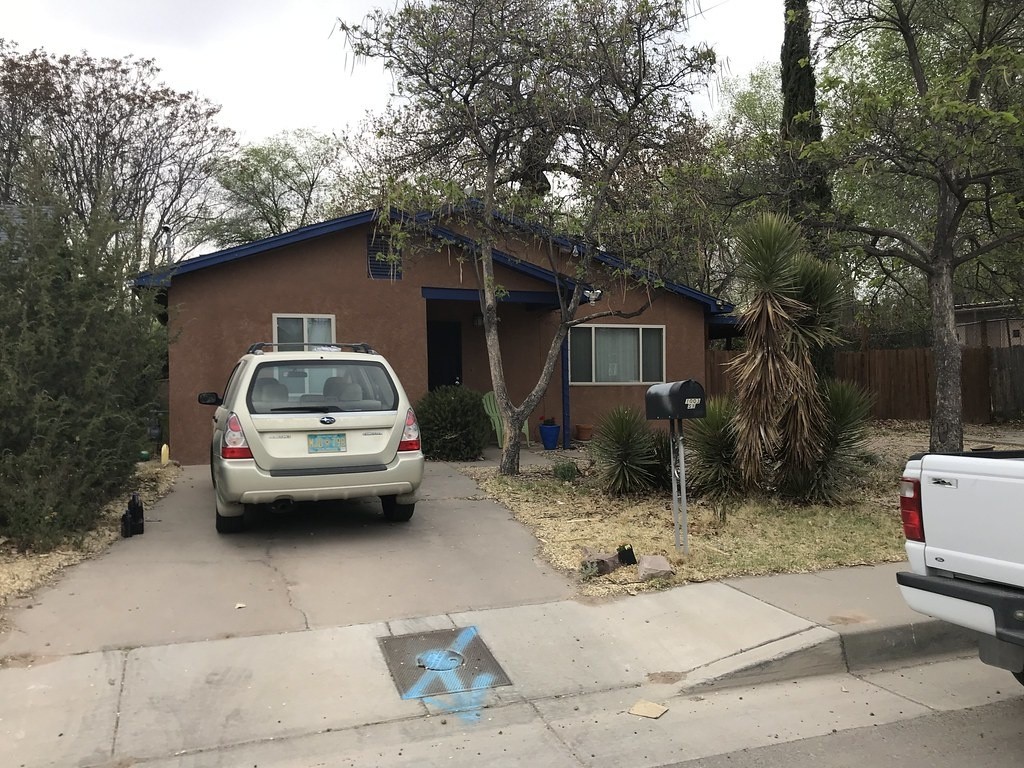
[895,449,1024,687]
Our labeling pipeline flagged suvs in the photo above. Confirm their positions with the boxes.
[198,341,424,535]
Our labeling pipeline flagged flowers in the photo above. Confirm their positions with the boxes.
[539,416,556,425]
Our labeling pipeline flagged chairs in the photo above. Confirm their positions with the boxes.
[324,377,363,401]
[482,390,531,449]
[250,378,288,403]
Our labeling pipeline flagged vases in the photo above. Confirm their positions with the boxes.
[540,426,560,450]
[576,424,592,440]
